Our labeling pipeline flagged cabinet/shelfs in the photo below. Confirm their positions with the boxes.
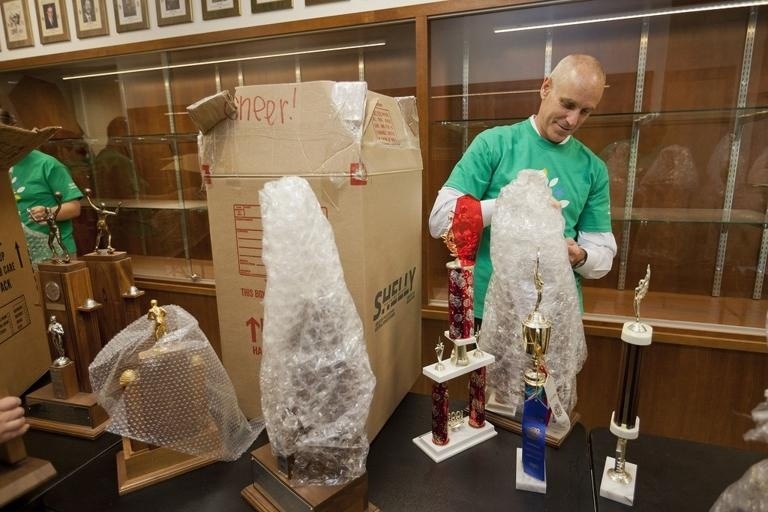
[423,0,765,448]
[3,7,421,356]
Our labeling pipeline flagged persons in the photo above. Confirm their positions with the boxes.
[0,107,85,260]
[0,395,30,444]
[45,4,57,29]
[94,115,149,254]
[427,53,617,350]
[83,0,95,22]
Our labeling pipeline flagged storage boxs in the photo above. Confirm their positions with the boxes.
[1,122,54,396]
[184,84,422,446]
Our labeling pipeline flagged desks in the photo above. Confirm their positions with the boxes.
[587,427,767,509]
[26,392,595,508]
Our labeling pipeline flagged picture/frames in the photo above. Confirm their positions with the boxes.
[73,2,109,37]
[34,1,70,44]
[250,1,295,13]
[2,1,33,48]
[113,1,150,32]
[155,2,193,26]
[203,0,240,21]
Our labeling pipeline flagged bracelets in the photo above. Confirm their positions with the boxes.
[573,254,587,271]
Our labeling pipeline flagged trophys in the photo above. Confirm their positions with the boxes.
[597,263,654,506]
[514,247,553,493]
[82,187,145,347]
[19,191,120,443]
[116,297,229,496]
[411,202,498,464]
